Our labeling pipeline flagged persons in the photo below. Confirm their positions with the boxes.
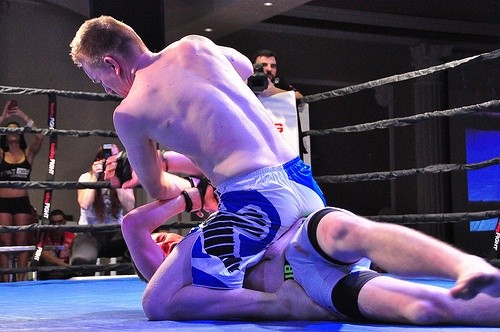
[63,143,136,278]
[107,149,500,326]
[253,47,306,107]
[40,208,76,279]
[67,16,340,321]
[0,101,44,283]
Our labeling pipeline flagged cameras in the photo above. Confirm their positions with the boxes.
[247,63,268,91]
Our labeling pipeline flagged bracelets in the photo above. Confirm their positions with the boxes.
[26,117,34,128]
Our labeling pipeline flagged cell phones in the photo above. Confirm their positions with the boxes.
[8,100,17,110]
